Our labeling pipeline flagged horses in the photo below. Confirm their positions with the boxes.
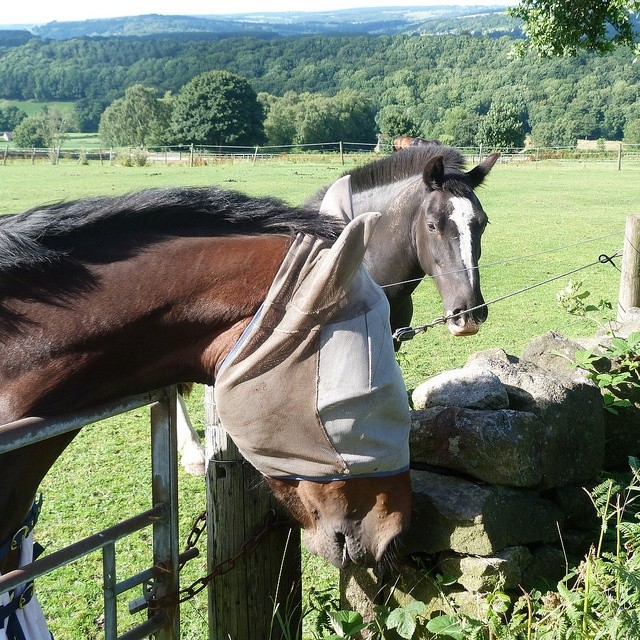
[0,184,414,640]
[392,136,442,151]
[147,143,502,477]
